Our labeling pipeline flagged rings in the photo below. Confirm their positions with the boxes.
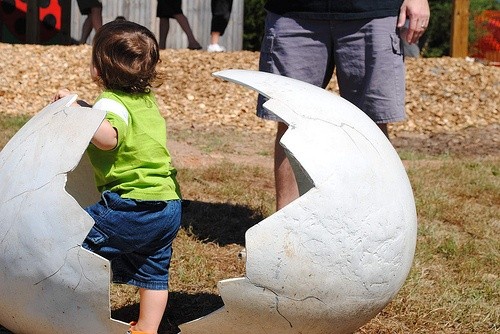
[420,24,427,29]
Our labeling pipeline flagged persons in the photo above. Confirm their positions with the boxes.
[78,0,103,45]
[52,20,182,334]
[256,0,430,213]
[156,0,202,50]
[400,11,422,58]
[207,0,234,52]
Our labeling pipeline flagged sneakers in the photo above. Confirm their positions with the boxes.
[207,43,228,52]
